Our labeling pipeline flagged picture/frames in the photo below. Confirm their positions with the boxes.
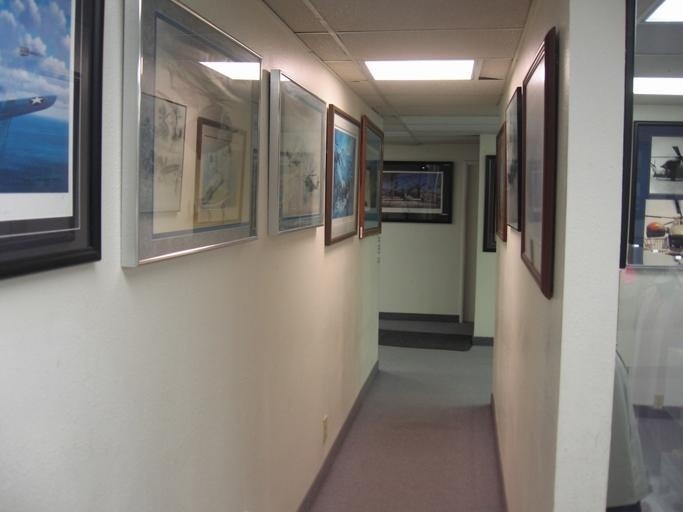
[268,66,328,240]
[117,0,266,271]
[626,118,683,272]
[383,158,454,224]
[481,25,559,302]
[323,100,359,249]
[0,1,108,282]
[356,111,385,240]
